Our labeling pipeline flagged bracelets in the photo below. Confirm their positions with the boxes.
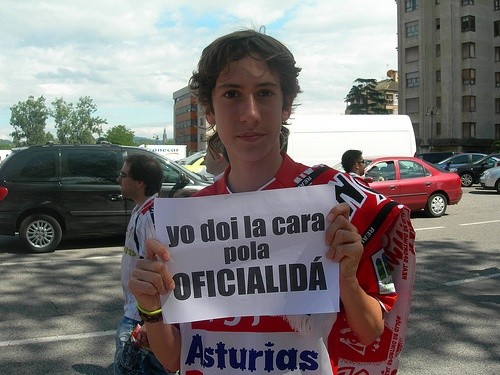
[135,304,163,324]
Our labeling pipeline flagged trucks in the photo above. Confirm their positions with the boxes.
[258,113,421,178]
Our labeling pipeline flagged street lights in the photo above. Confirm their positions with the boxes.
[425,104,440,154]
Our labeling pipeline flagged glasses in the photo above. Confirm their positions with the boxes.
[358,159,365,165]
[119,171,136,179]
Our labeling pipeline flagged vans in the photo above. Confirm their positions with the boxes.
[0,143,217,254]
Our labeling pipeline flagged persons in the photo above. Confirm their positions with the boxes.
[111,153,168,375]
[204,133,231,181]
[342,149,366,178]
[125,29,398,375]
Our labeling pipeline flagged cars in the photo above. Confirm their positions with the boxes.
[171,150,216,183]
[333,156,463,221]
[435,153,497,186]
[481,164,500,192]
